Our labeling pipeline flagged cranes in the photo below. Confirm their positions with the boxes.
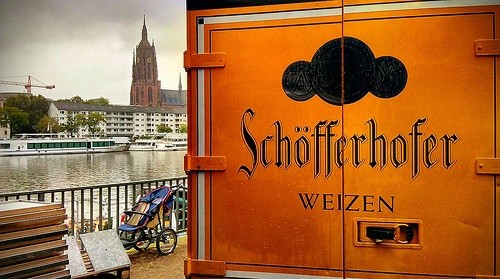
[0,74,55,93]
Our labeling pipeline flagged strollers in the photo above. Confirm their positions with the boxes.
[117,184,181,255]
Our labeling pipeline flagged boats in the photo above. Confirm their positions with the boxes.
[0,136,130,157]
[130,131,188,153]
